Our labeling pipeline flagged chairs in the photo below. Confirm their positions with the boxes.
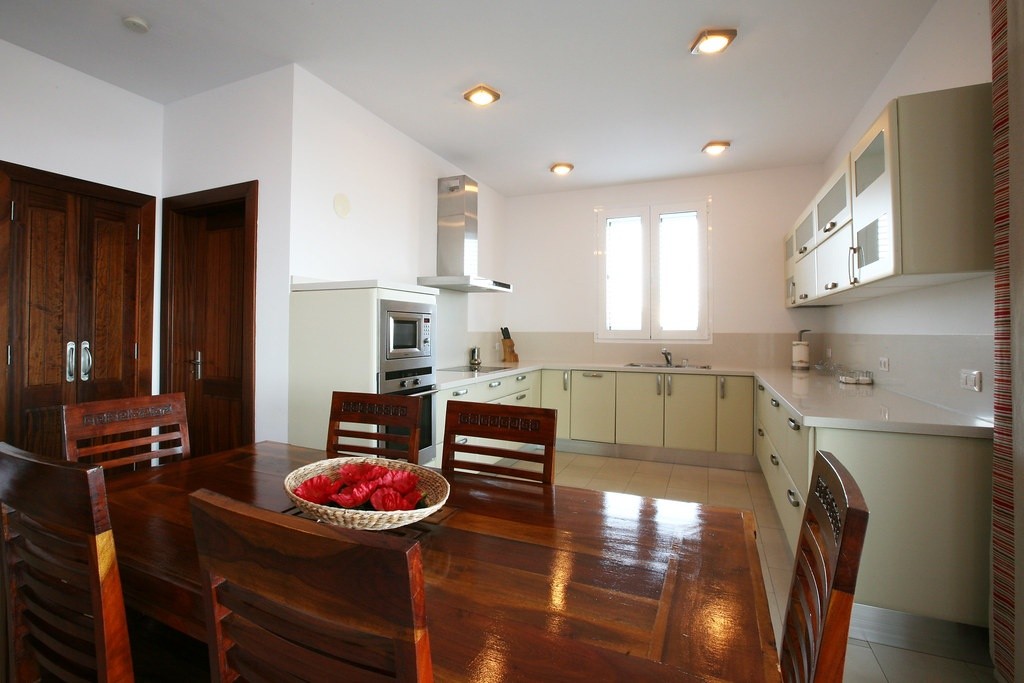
[189,486,434,683]
[1,440,136,682]
[58,391,192,470]
[776,450,870,683]
[441,399,559,484]
[327,390,424,465]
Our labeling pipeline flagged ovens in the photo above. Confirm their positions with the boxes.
[377,356,437,465]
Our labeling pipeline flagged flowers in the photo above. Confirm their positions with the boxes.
[293,461,428,511]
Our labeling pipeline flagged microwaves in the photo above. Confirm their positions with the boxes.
[379,299,438,360]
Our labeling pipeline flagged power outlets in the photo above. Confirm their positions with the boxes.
[878,356,889,371]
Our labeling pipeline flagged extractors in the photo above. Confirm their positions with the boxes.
[417,175,514,293]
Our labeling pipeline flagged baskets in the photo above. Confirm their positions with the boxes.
[283,456,451,532]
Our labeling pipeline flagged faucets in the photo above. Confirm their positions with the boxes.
[660,349,672,365]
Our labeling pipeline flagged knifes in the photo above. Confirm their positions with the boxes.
[501,327,511,339]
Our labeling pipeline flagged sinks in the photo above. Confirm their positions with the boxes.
[672,364,711,371]
[624,363,667,370]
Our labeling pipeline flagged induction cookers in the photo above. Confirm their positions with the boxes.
[436,365,512,373]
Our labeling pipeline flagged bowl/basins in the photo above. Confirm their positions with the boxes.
[284,457,450,530]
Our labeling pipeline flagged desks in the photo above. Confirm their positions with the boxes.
[20,441,784,683]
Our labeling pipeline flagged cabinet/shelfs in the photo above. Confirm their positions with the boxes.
[435,368,992,631]
[782,81,995,309]
